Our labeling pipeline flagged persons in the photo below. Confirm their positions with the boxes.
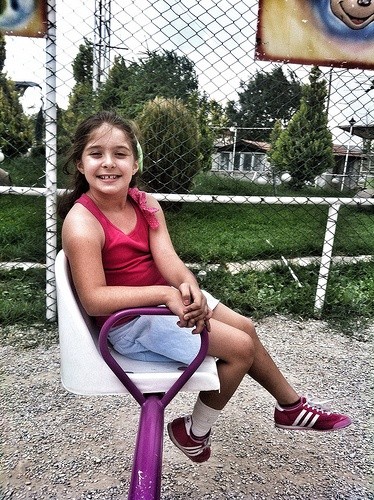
[61,111,352,463]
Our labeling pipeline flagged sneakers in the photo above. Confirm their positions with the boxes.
[167,415,211,463]
[273,397,352,432]
